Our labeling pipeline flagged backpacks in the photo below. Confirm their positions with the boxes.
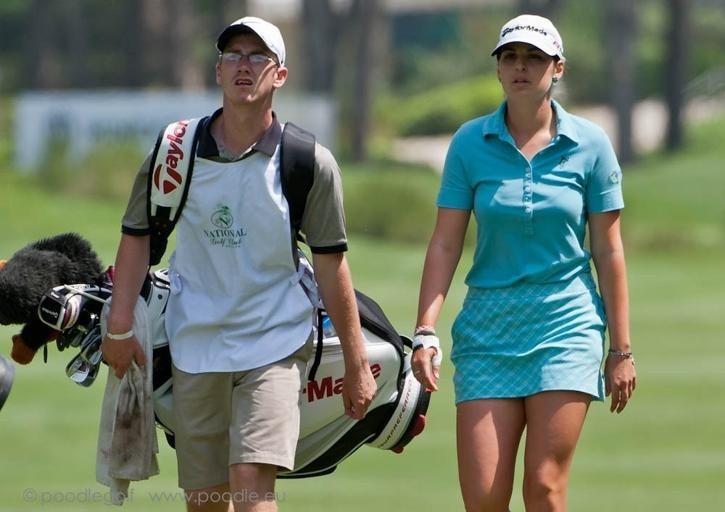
[113,109,433,484]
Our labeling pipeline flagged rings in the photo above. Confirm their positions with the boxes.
[413,370,421,376]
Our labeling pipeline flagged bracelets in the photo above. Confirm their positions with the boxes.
[415,323,437,335]
[607,348,638,361]
[104,329,137,339]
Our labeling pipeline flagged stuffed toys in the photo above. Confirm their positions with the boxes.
[1,230,106,364]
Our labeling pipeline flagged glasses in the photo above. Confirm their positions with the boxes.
[216,50,280,69]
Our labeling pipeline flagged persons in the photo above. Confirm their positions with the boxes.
[99,14,380,511]
[409,11,638,512]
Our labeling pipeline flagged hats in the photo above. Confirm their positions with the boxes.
[213,13,288,70]
[486,13,567,62]
